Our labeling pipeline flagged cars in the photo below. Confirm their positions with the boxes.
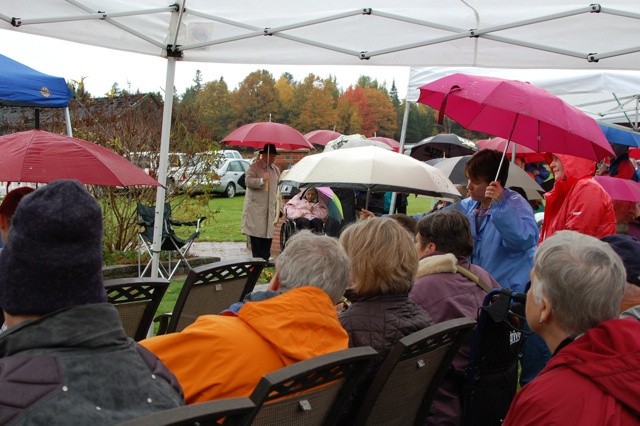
[149,152,192,187]
[116,151,155,189]
[182,158,250,197]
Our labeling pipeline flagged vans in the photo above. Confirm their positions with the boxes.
[173,149,243,194]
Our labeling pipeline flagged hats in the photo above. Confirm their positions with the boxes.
[600,236,639,287]
[0,180,109,315]
[259,144,280,154]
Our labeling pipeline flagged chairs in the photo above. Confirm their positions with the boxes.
[153,257,267,342]
[113,397,256,425]
[347,315,477,426]
[103,277,170,343]
[239,345,379,426]
[137,201,206,283]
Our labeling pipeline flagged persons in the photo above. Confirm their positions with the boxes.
[394,192,409,214]
[502,230,640,426]
[336,217,437,389]
[135,227,354,426]
[240,143,281,266]
[537,151,616,248]
[509,156,535,181]
[358,148,554,420]
[408,209,503,425]
[0,177,188,425]
[283,186,329,234]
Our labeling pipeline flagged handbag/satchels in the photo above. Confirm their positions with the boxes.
[414,287,527,426]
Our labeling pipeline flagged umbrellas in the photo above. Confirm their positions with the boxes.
[276,144,463,211]
[219,112,317,191]
[592,173,640,203]
[304,125,407,153]
[0,107,169,191]
[315,186,344,224]
[415,73,617,182]
[481,137,546,157]
[408,132,480,162]
[595,118,639,148]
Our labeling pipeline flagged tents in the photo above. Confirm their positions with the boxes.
[388,66,640,215]
[1,0,640,283]
[0,54,74,147]
